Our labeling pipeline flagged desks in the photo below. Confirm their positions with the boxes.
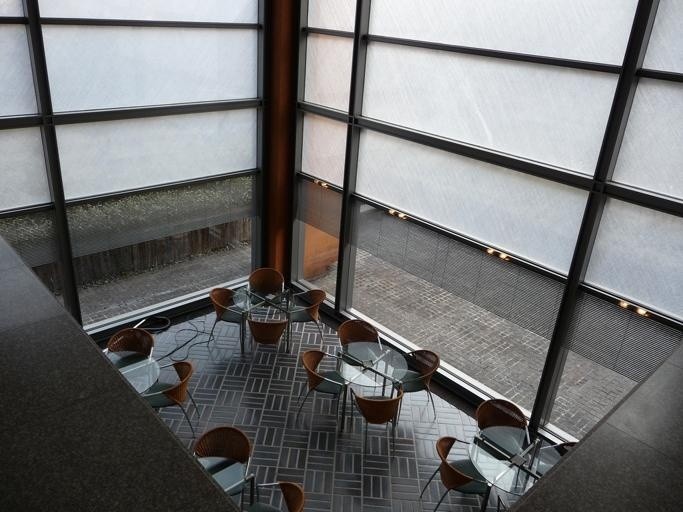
[334,341,407,431]
[105,352,161,396]
[233,285,298,354]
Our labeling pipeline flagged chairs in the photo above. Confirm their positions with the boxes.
[291,290,326,342]
[244,482,309,512]
[141,362,200,436]
[247,318,288,369]
[338,320,384,389]
[390,350,439,423]
[476,399,530,491]
[207,288,247,357]
[420,437,491,511]
[468,427,561,496]
[350,384,403,458]
[248,270,285,319]
[108,329,155,388]
[294,350,343,423]
[496,440,579,511]
[192,425,254,507]
